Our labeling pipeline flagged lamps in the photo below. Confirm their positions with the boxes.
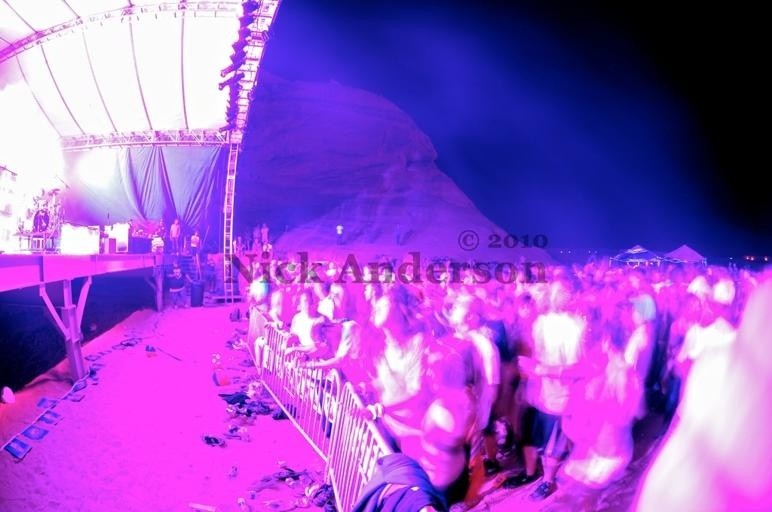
[216,0,260,135]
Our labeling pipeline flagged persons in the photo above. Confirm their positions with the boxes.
[157,219,167,241]
[170,219,180,256]
[190,230,200,258]
[168,259,192,310]
